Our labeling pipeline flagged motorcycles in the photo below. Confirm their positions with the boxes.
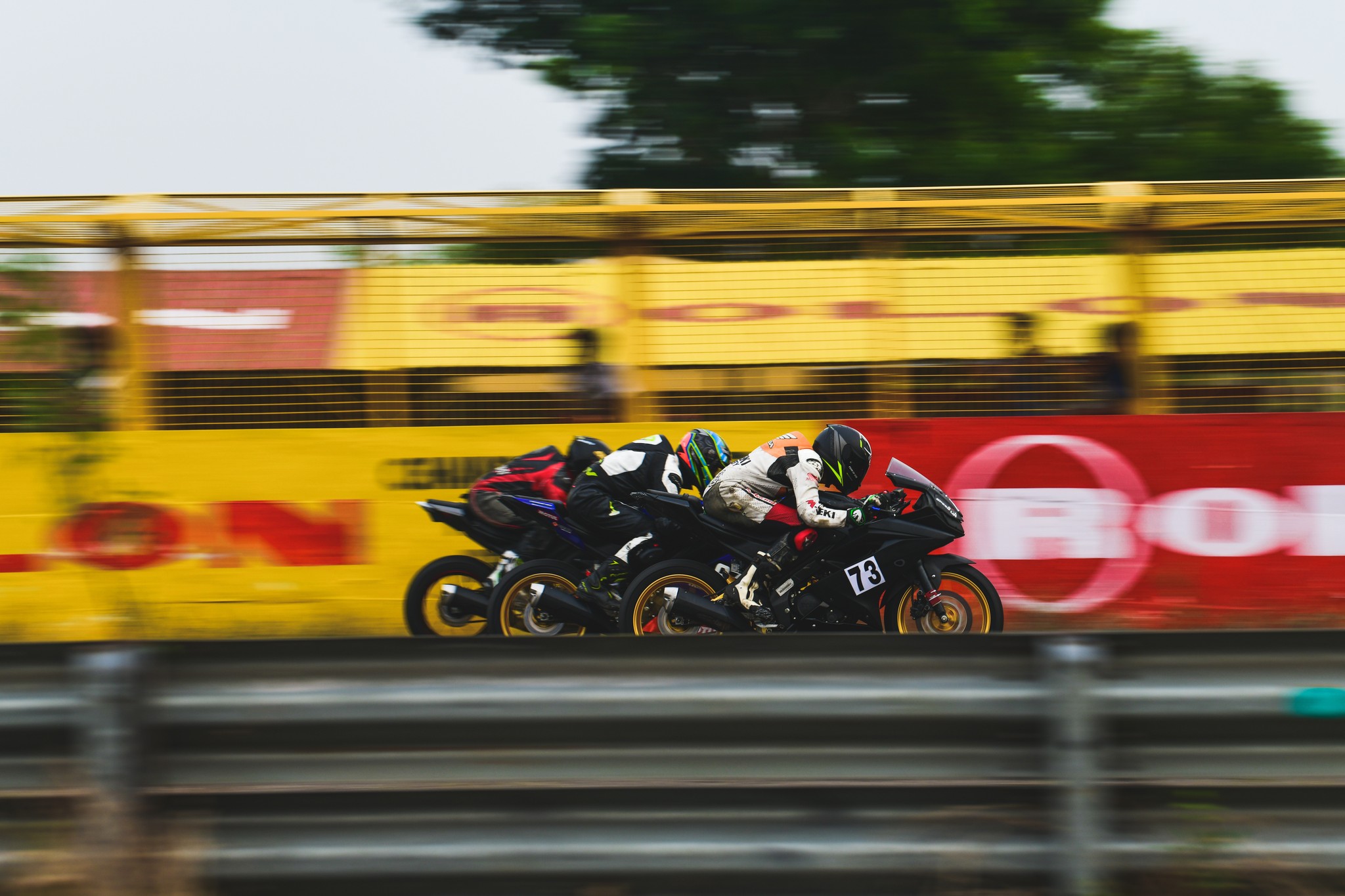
[617,456,1004,635]
[402,489,712,639]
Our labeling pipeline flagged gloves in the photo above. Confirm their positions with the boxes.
[862,492,890,519]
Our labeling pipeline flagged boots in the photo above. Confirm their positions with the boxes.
[576,555,629,611]
[484,549,526,598]
[723,551,781,623]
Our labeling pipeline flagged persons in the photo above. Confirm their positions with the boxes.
[998,304,1049,407]
[54,316,125,405]
[566,430,731,620]
[697,425,909,624]
[464,435,611,601]
[559,324,637,420]
[1094,317,1143,403]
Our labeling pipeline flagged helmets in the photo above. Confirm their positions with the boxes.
[677,428,731,491]
[813,424,873,495]
[567,435,611,469]
